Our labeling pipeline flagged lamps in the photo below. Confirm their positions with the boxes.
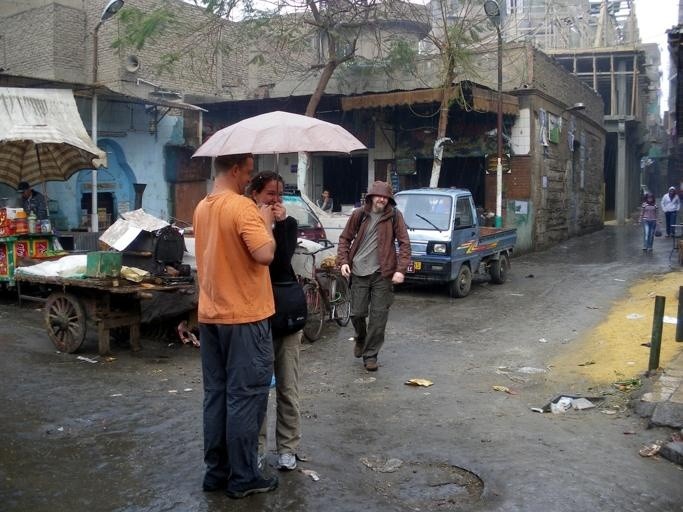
[148,118,157,135]
[564,103,585,113]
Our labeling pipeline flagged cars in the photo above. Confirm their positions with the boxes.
[281,195,326,244]
[662,178,683,196]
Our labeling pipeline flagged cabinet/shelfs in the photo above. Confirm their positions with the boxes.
[0,232,55,306]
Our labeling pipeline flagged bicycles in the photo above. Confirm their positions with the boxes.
[293,239,352,342]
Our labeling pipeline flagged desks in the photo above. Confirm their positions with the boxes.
[54,230,100,252]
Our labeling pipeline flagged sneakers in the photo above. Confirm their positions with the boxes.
[203,474,231,493]
[364,357,378,372]
[664,233,674,237]
[642,246,653,251]
[354,337,364,358]
[274,451,298,471]
[223,472,279,500]
[257,453,266,472]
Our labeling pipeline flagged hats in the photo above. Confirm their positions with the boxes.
[16,181,30,193]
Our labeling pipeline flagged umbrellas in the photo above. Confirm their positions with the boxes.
[189,109,370,204]
[0,120,100,218]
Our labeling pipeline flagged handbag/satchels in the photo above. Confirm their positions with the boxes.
[270,279,307,315]
[655,224,662,237]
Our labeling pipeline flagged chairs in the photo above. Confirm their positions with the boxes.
[57,229,88,233]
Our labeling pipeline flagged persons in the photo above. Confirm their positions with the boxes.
[13,181,45,217]
[660,186,682,238]
[314,188,334,213]
[637,192,659,251]
[335,180,413,372]
[246,169,307,472]
[190,152,278,500]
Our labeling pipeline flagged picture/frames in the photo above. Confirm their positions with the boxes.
[548,112,560,144]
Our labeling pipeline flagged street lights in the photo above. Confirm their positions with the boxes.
[483,0,505,233]
[90,0,124,232]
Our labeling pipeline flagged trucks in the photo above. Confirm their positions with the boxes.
[393,186,517,298]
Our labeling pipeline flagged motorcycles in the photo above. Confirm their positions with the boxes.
[641,184,651,200]
